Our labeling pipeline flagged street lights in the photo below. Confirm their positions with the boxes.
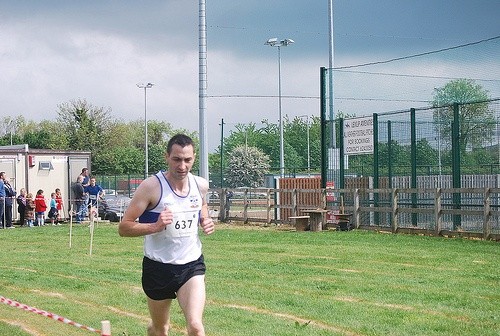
[264,37,295,178]
[136,83,154,180]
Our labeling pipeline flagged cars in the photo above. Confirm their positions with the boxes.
[97,188,133,222]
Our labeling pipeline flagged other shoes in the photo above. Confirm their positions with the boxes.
[0,225,3,229]
[7,226,15,229]
[52,224,56,226]
[57,222,61,225]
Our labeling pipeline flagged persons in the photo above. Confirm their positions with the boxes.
[80,168,106,218]
[118,134,215,336]
[17,188,28,227]
[0,172,17,228]
[50,188,64,226]
[73,176,88,221]
[24,190,49,227]
